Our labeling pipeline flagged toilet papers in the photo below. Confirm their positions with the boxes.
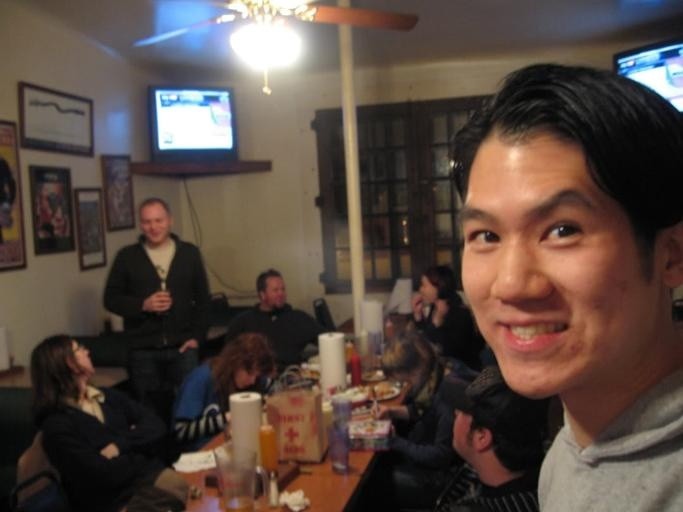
[385,279,413,317]
[358,299,385,344]
[228,392,263,467]
[317,332,347,397]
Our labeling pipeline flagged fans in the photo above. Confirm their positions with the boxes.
[123,0,422,47]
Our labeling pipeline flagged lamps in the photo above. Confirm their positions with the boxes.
[225,13,311,95]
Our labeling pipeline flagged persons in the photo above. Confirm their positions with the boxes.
[29,333,168,511]
[0,156,17,243]
[33,168,69,240]
[369,265,485,464]
[176,265,321,442]
[448,64,682,511]
[102,199,211,413]
[433,367,546,512]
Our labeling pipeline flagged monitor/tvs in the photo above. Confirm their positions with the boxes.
[613,36,683,117]
[148,85,237,162]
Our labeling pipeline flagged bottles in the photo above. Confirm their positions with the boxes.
[384,319,395,340]
[301,362,320,374]
[344,339,361,386]
[267,469,280,506]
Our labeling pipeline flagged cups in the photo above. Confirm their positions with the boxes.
[211,445,269,512]
[324,397,353,474]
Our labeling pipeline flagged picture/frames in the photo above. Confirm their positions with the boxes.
[101,155,137,231]
[27,165,79,255]
[15,81,97,158]
[72,188,108,270]
[0,117,28,276]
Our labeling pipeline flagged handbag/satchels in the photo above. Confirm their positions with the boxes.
[266,390,324,463]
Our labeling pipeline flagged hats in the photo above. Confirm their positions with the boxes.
[439,364,550,428]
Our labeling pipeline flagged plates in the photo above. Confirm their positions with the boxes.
[335,367,399,408]
